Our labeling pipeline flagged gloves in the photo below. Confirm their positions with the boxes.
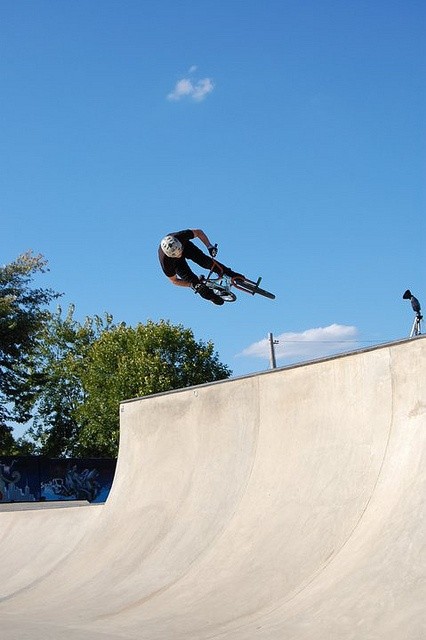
[206,244,218,257]
[189,281,205,293]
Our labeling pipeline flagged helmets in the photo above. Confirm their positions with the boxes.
[161,236,182,258]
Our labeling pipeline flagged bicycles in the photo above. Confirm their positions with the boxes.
[191,243,276,302]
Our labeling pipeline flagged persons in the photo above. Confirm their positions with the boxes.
[157,228,246,305]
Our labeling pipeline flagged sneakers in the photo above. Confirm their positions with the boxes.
[210,293,224,306]
[233,272,245,283]
